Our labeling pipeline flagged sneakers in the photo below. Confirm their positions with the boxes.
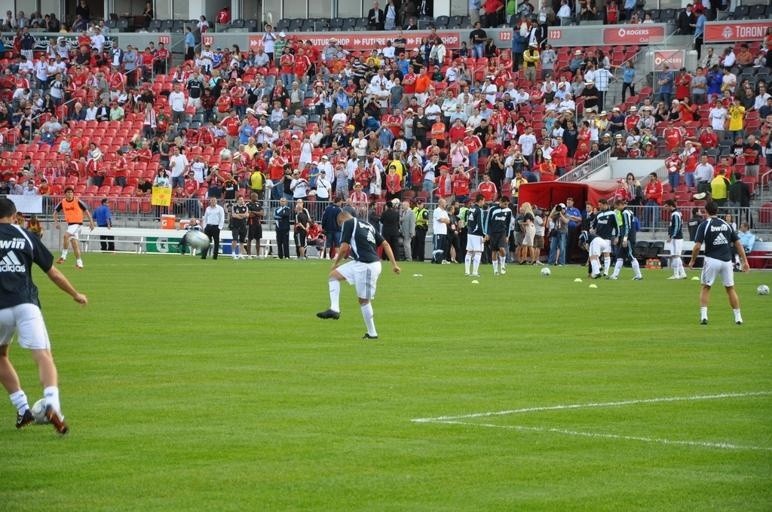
[464,272,472,276]
[316,308,342,319]
[735,317,743,323]
[588,265,608,279]
[606,276,617,280]
[471,272,481,277]
[56,257,65,264]
[633,276,643,280]
[501,263,507,274]
[362,333,377,340]
[699,317,708,325]
[494,271,499,275]
[666,275,680,279]
[678,273,687,279]
[15,409,35,429]
[75,259,83,268]
[537,261,544,266]
[45,400,66,434]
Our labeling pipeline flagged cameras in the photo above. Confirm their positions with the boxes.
[556,205,562,211]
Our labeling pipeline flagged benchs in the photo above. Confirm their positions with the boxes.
[79,226,434,260]
[657,239,772,270]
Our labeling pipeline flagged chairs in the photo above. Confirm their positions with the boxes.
[631,240,670,266]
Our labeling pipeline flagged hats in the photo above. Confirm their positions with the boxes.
[0,0,693,190]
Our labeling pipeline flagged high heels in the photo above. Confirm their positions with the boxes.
[516,260,536,266]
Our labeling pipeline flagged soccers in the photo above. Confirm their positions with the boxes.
[185,232,210,249]
[30,397,49,424]
[541,266,551,276]
[757,284,770,295]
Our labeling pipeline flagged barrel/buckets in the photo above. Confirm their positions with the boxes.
[179,220,191,229]
[221,238,239,254]
[161,213,176,229]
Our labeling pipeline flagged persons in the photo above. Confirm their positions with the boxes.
[687,202,749,325]
[0,0,772,279]
[0,198,88,434]
[316,211,401,340]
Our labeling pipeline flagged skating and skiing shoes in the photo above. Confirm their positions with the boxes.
[181,250,338,260]
